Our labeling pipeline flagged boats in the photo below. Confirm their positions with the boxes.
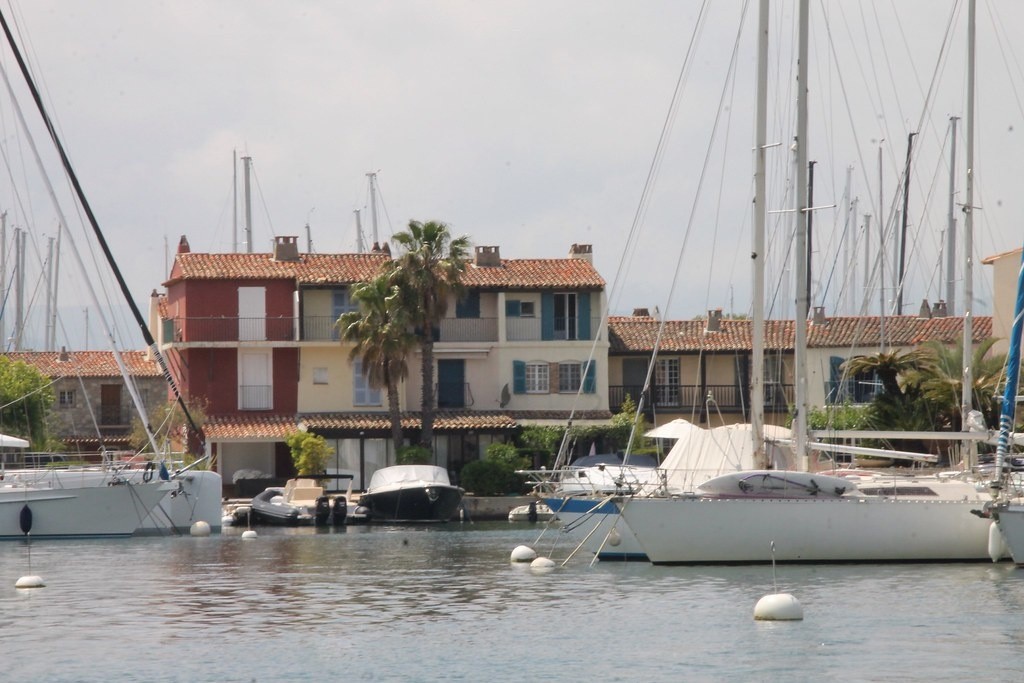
[252,490,371,525]
[509,503,558,521]
[359,466,465,521]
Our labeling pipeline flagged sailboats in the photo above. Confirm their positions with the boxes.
[0,1,224,536]
[514,0,1022,568]
